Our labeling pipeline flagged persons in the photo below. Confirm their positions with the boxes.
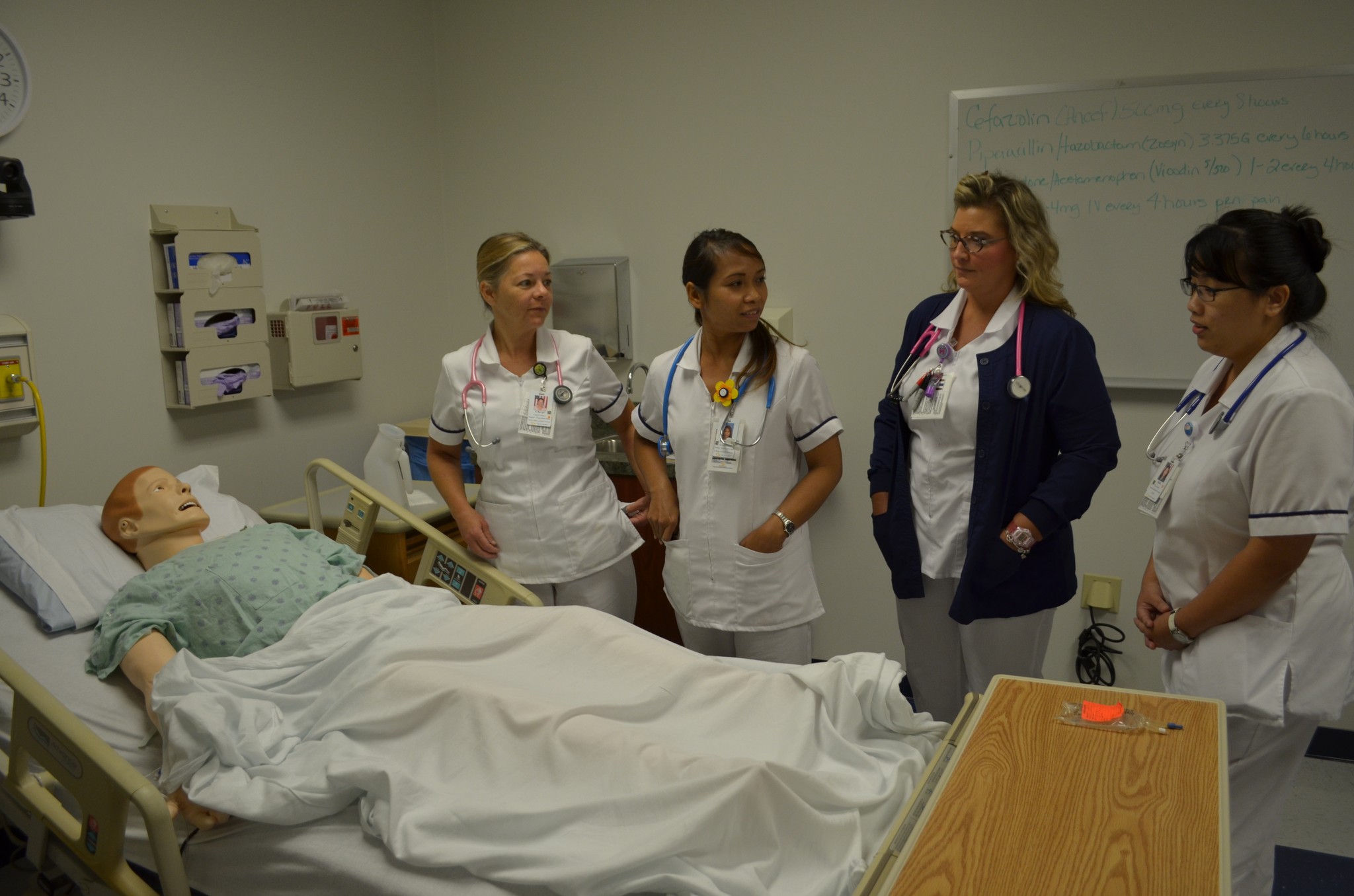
[1134,199,1354,896]
[864,169,1122,726]
[628,225,845,666]
[86,462,935,866]
[424,230,651,634]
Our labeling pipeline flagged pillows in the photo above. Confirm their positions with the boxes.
[0,461,259,634]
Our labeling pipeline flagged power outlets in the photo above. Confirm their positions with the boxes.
[1080,573,1122,614]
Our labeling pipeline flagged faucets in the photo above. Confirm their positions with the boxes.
[626,362,649,394]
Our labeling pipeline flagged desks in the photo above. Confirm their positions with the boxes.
[857,672,1243,896]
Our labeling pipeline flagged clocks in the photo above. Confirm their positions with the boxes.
[0,26,31,140]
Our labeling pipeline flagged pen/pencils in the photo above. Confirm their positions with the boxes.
[1208,411,1225,434]
[901,372,928,401]
[911,376,929,410]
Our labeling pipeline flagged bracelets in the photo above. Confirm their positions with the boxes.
[1002,526,1031,559]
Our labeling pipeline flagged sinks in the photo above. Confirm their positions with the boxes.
[590,433,628,455]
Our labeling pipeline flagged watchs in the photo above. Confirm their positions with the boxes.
[1003,518,1039,555]
[1166,606,1195,645]
[771,508,795,538]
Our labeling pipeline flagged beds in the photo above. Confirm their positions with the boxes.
[0,495,948,896]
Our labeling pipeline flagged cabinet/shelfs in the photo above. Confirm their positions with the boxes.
[151,200,277,412]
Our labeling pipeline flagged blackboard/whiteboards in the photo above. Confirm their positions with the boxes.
[949,67,1354,392]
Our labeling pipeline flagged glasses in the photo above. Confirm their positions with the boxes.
[1180,277,1252,302]
[939,229,1009,254]
